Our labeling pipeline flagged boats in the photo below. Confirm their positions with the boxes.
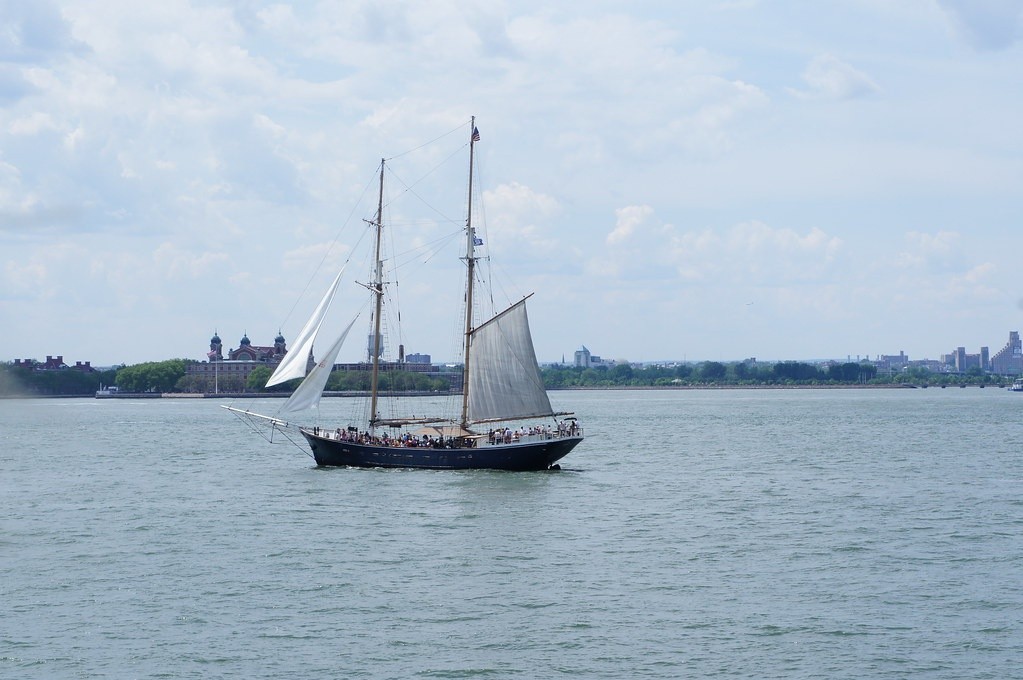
[1008,377,1023,392]
[161,391,206,398]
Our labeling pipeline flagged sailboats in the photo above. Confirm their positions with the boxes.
[217,116,587,473]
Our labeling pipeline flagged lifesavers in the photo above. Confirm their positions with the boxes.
[363,462,371,468]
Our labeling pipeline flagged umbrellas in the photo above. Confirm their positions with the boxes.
[564,417,578,421]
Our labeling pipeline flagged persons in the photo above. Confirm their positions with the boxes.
[336,418,580,448]
